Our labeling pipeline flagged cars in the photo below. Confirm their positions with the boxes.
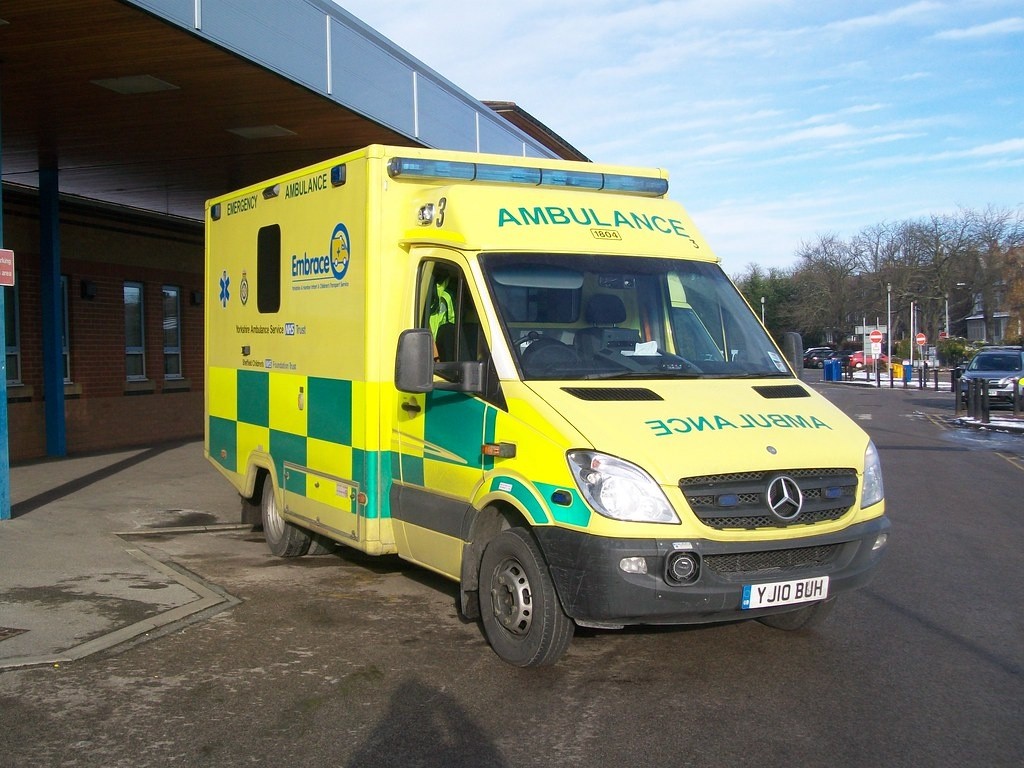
[850,351,888,368]
[956,350,1024,411]
[803,347,854,368]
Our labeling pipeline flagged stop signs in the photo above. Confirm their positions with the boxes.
[939,333,946,342]
[870,330,882,343]
[916,333,925,345]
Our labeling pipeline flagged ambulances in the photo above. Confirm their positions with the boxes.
[202,143,890,670]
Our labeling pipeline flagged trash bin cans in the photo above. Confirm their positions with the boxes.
[823,358,842,382]
[903,364,912,381]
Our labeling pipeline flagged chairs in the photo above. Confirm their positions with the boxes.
[434,298,518,399]
[573,295,641,379]
[980,357,995,370]
[1003,358,1018,371]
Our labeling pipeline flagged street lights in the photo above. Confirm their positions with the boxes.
[886,282,891,378]
[761,296,764,324]
